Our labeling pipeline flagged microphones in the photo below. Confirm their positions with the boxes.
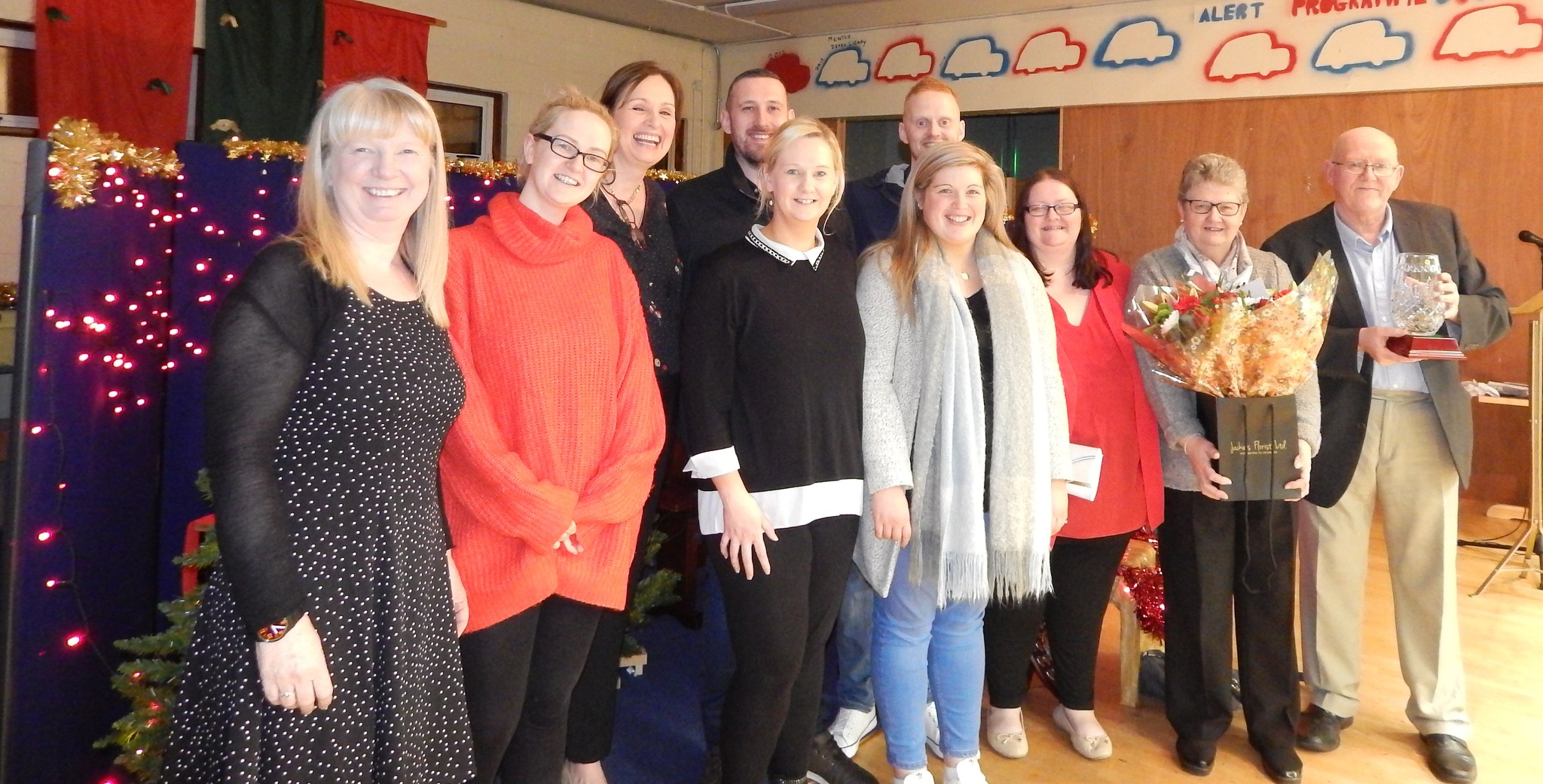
[1519,230,1543,248]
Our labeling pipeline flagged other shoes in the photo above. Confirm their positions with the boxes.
[903,769,935,784]
[986,704,1028,758]
[943,757,988,784]
[1053,703,1112,760]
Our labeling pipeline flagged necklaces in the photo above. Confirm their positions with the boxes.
[956,256,975,280]
[604,176,641,205]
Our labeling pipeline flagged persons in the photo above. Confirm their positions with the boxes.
[156,79,483,784]
[435,59,1512,784]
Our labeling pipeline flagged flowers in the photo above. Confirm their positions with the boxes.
[1143,275,1294,351]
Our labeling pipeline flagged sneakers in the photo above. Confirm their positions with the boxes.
[925,701,981,760]
[825,704,877,758]
[805,729,879,784]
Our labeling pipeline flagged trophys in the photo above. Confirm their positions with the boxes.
[1384,253,1468,360]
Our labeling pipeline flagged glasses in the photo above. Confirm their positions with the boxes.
[535,131,611,174]
[1025,202,1080,216]
[1183,198,1245,216]
[1331,160,1400,177]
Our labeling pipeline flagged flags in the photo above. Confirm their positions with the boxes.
[193,1,439,151]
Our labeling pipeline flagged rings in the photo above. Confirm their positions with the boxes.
[279,689,295,699]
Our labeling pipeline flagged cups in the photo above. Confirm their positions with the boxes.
[1391,252,1446,336]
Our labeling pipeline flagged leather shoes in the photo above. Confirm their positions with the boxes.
[1176,737,1216,776]
[1296,702,1355,752]
[1252,739,1303,784]
[1419,731,1476,784]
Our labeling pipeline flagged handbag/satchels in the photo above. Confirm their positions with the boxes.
[1194,391,1303,595]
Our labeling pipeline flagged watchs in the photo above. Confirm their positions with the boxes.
[254,602,307,643]
